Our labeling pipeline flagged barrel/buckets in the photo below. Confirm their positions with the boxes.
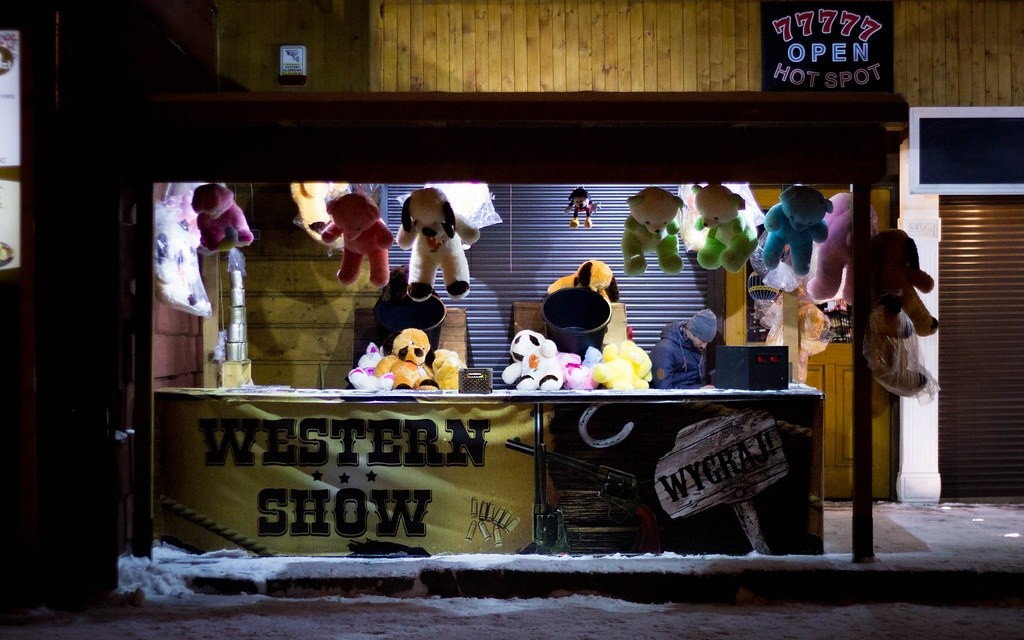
[375,290,447,363]
[541,286,613,357]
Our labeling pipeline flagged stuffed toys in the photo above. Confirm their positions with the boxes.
[690,184,758,273]
[763,185,938,396]
[287,183,395,287]
[565,188,602,230]
[621,185,686,276]
[501,330,653,390]
[348,328,468,391]
[548,259,620,304]
[152,184,254,319]
[382,188,481,301]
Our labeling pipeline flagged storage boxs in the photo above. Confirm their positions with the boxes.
[458,367,493,395]
[714,345,789,391]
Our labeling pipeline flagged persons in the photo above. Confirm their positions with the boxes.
[649,309,718,391]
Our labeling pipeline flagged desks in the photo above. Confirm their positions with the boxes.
[150,381,825,555]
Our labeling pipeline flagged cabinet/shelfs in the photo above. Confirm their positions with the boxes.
[708,185,892,501]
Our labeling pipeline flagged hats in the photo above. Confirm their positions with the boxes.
[686,308,717,343]
